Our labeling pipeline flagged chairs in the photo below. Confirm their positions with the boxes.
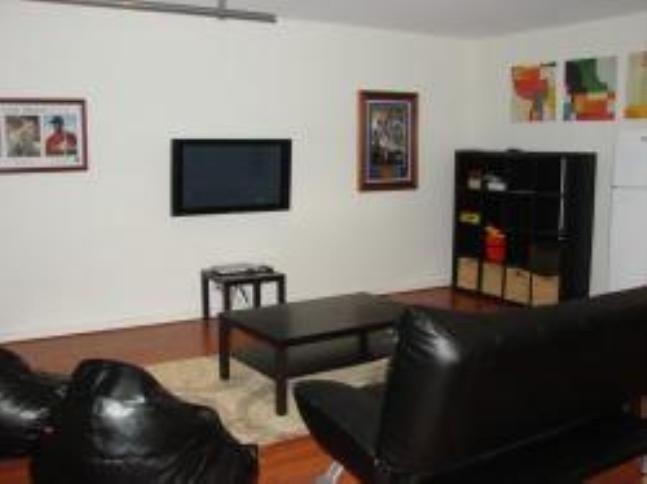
[1,346,259,484]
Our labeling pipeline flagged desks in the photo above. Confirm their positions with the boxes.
[219,293,414,416]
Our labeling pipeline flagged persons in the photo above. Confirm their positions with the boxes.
[4,116,33,157]
[45,115,77,157]
[19,115,41,142]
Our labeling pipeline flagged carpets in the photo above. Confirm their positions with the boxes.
[136,313,461,447]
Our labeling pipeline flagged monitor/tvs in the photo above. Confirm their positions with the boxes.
[171,137,293,217]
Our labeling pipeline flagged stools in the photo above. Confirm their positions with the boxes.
[200,262,287,321]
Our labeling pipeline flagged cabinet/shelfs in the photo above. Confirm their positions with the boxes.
[452,150,596,307]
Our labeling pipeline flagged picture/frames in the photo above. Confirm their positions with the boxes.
[358,89,418,189]
[0,95,87,171]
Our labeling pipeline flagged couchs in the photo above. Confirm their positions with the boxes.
[289,284,646,484]
